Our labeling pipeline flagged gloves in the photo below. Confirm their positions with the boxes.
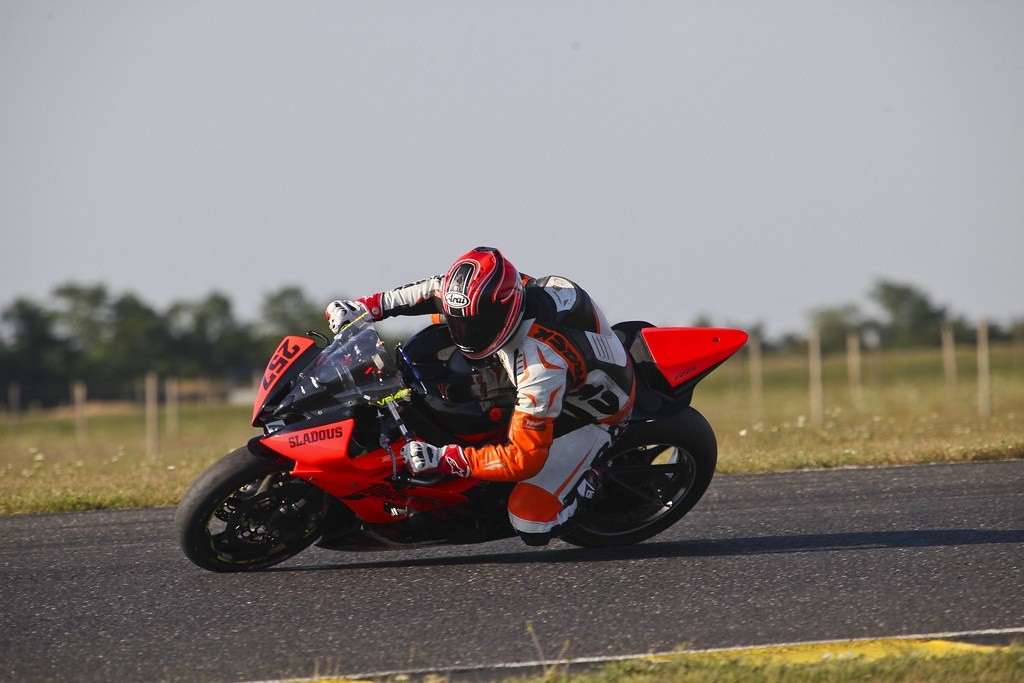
[400,441,471,480]
[324,289,383,333]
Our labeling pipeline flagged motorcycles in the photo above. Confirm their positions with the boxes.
[173,311,749,575]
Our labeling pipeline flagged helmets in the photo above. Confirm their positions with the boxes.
[442,246,527,360]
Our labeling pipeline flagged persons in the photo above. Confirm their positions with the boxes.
[325,246,638,548]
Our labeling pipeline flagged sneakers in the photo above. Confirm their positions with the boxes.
[589,466,606,493]
